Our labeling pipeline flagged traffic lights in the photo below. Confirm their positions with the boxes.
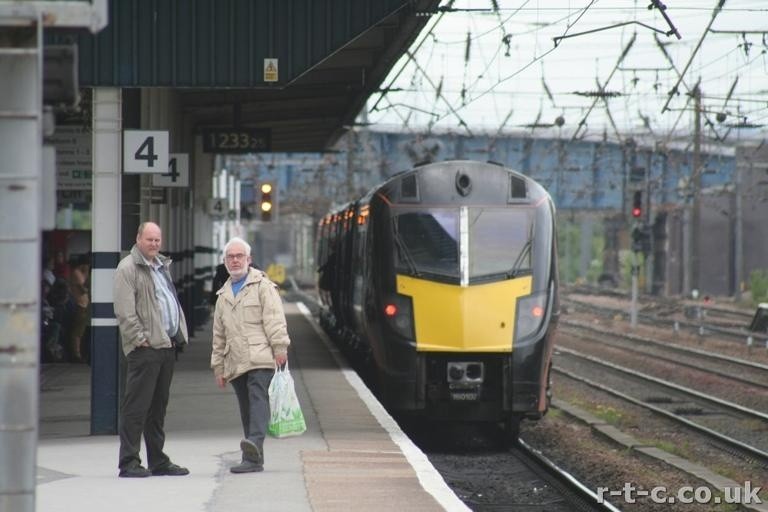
[631,189,644,217]
[258,180,274,219]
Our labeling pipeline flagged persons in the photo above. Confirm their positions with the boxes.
[211,238,292,473]
[40,249,90,364]
[317,236,341,333]
[111,221,193,477]
[208,256,262,317]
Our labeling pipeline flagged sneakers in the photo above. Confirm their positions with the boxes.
[230,460,265,474]
[150,461,191,477]
[118,464,154,478]
[240,436,264,465]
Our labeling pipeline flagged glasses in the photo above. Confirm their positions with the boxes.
[224,254,248,260]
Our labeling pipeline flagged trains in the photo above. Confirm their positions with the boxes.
[313,158,563,442]
